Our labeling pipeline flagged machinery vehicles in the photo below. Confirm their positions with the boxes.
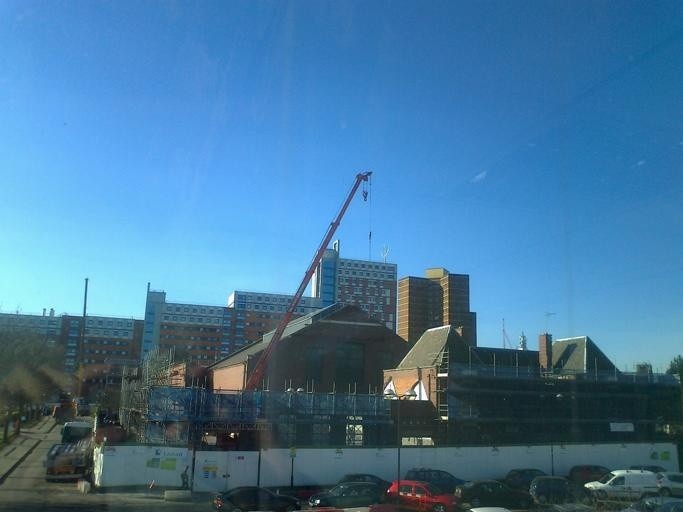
[74,275,89,405]
[201,169,376,450]
[53,393,77,423]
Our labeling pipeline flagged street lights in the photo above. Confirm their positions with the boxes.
[284,382,304,484]
[381,388,418,481]
[549,387,567,476]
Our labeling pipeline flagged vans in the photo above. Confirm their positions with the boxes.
[60,422,94,445]
[621,496,683,512]
[584,469,660,501]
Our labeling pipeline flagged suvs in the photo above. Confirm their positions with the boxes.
[405,469,466,492]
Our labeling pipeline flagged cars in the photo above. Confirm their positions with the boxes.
[656,471,683,496]
[386,478,456,512]
[546,503,593,512]
[308,482,381,507]
[466,506,509,512]
[211,485,301,512]
[631,465,665,473]
[454,479,532,510]
[76,403,90,416]
[570,465,611,482]
[45,445,88,482]
[339,473,392,493]
[506,468,548,489]
[529,476,589,502]
